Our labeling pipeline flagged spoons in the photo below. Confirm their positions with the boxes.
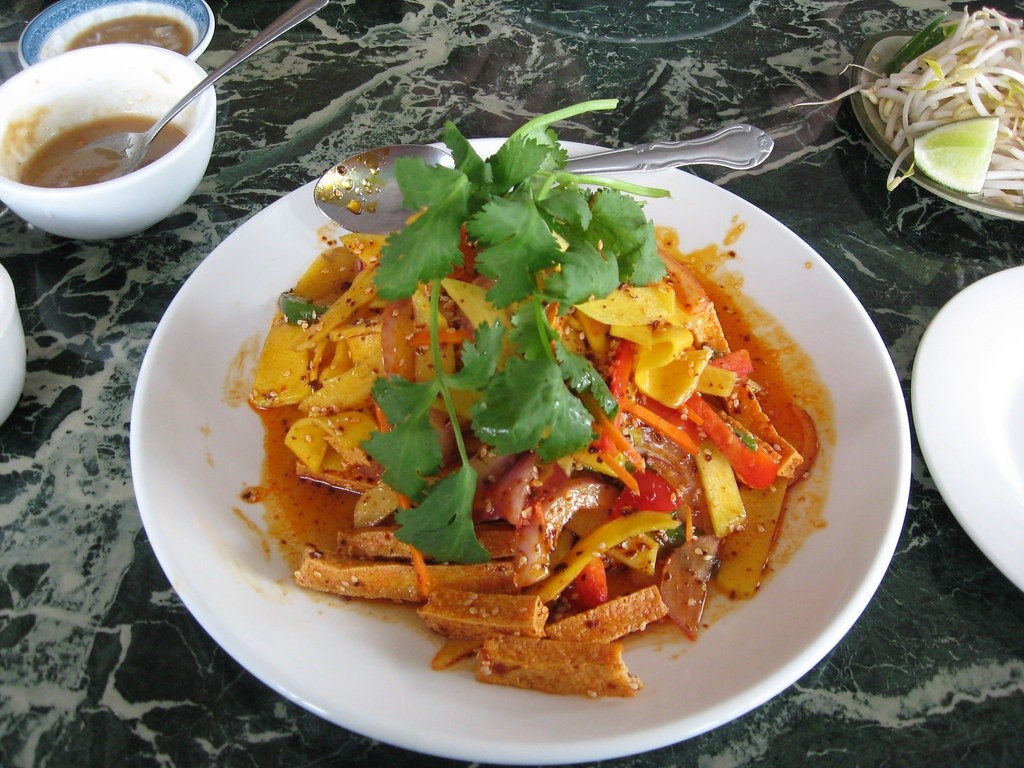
[314,126,774,233]
[75,0,330,173]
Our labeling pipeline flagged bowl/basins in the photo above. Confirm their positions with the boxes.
[0,44,216,240]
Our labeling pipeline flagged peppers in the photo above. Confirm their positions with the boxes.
[279,291,329,324]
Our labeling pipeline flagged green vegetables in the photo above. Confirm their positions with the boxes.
[356,98,670,563]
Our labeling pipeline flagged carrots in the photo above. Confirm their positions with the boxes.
[374,285,780,605]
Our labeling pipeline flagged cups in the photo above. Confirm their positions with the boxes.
[0,263,25,428]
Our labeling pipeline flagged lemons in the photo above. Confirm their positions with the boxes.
[914,116,999,193]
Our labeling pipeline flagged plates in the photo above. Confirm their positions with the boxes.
[131,136,911,763]
[19,0,215,69]
[850,30,1024,221]
[911,263,1024,590]
